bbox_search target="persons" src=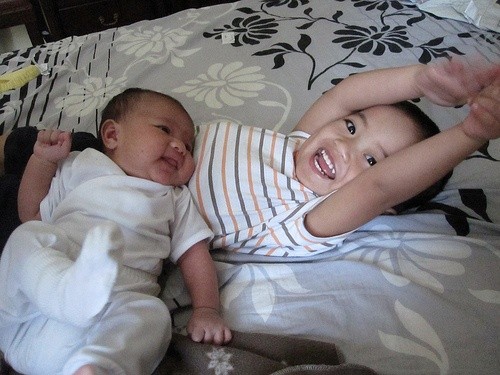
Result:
[0,61,500,256]
[0,88,232,375]
